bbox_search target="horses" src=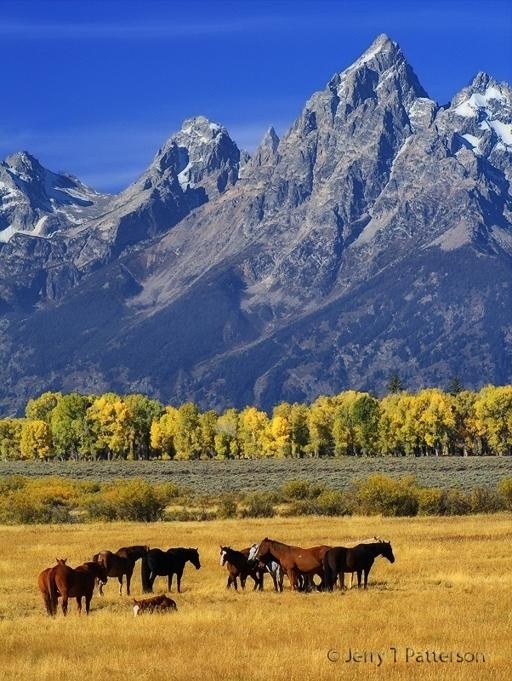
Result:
[130,595,179,618]
[93,544,147,597]
[38,556,69,614]
[46,560,109,617]
[218,535,396,591]
[139,546,201,593]
